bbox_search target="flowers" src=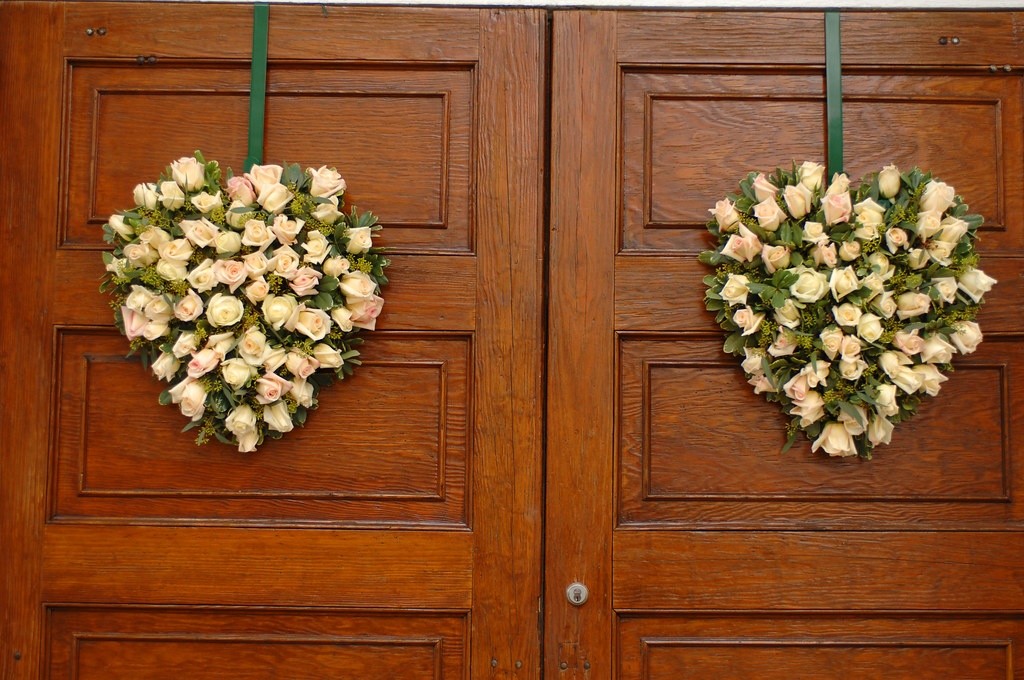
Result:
[699,161,1000,463]
[97,150,392,454]
